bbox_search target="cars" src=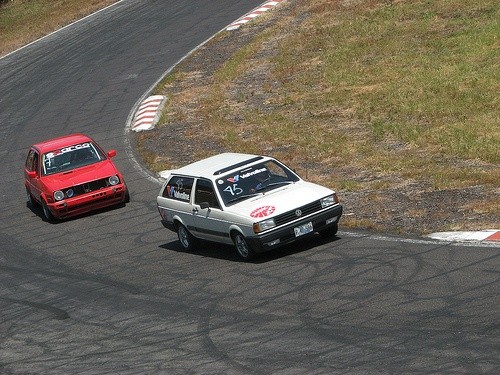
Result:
[157,152,343,262]
[24,133,130,223]
